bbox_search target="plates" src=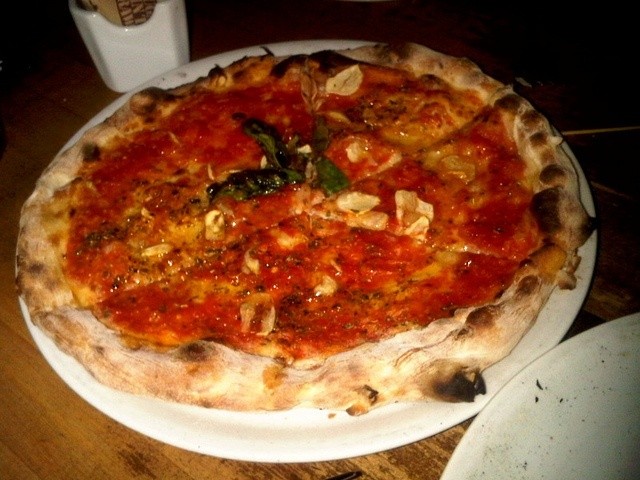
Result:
[440,312,640,480]
